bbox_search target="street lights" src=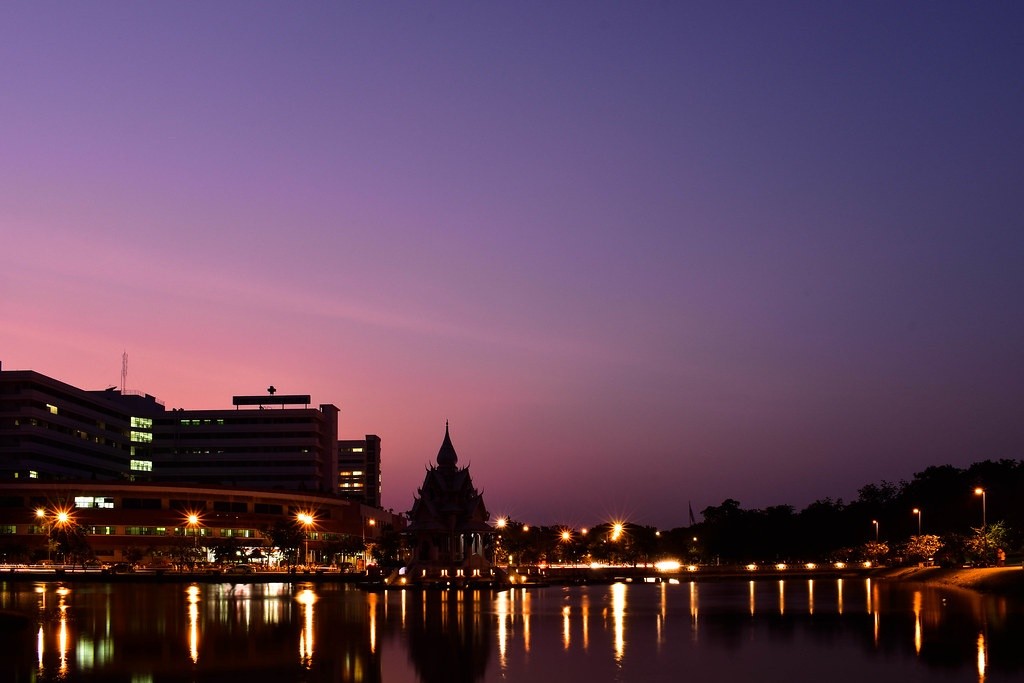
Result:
[872,520,879,542]
[303,515,314,564]
[913,506,922,538]
[973,488,986,529]
